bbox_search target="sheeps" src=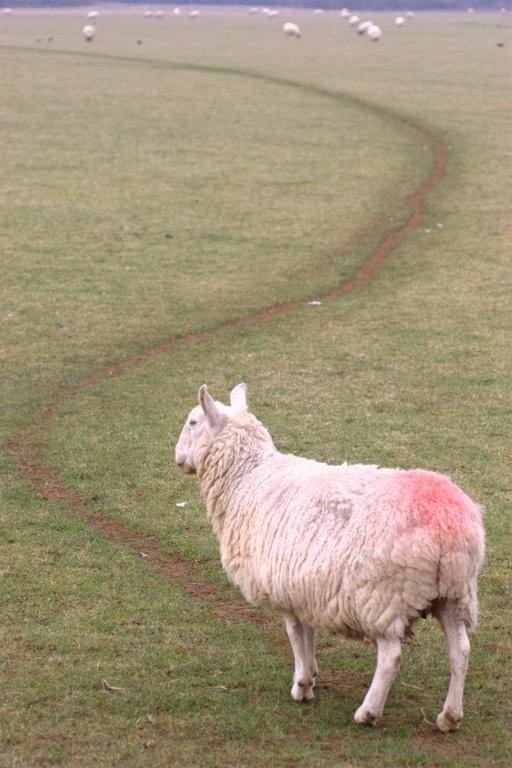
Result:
[394,17,406,26]
[247,7,278,18]
[1,7,12,14]
[173,8,180,17]
[407,12,414,19]
[173,382,487,733]
[142,11,152,18]
[339,7,382,42]
[155,10,164,18]
[284,22,302,39]
[311,7,323,16]
[87,11,99,20]
[190,10,199,18]
[83,25,96,40]
